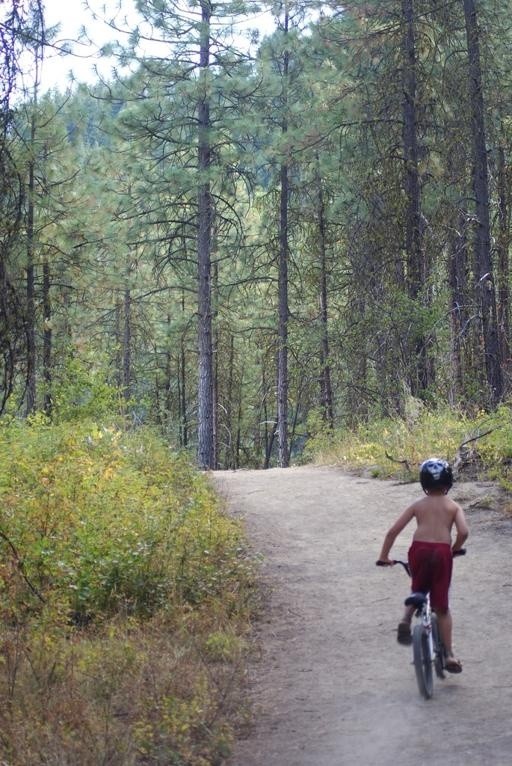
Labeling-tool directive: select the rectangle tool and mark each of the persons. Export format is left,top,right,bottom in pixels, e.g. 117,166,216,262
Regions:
379,457,469,674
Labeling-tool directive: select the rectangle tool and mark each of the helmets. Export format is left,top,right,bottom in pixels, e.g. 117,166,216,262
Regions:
419,458,453,491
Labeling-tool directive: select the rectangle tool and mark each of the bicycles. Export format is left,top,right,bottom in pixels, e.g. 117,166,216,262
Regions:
373,546,471,701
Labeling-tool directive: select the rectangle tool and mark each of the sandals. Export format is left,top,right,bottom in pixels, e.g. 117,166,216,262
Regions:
397,616,411,644
446,651,462,673
